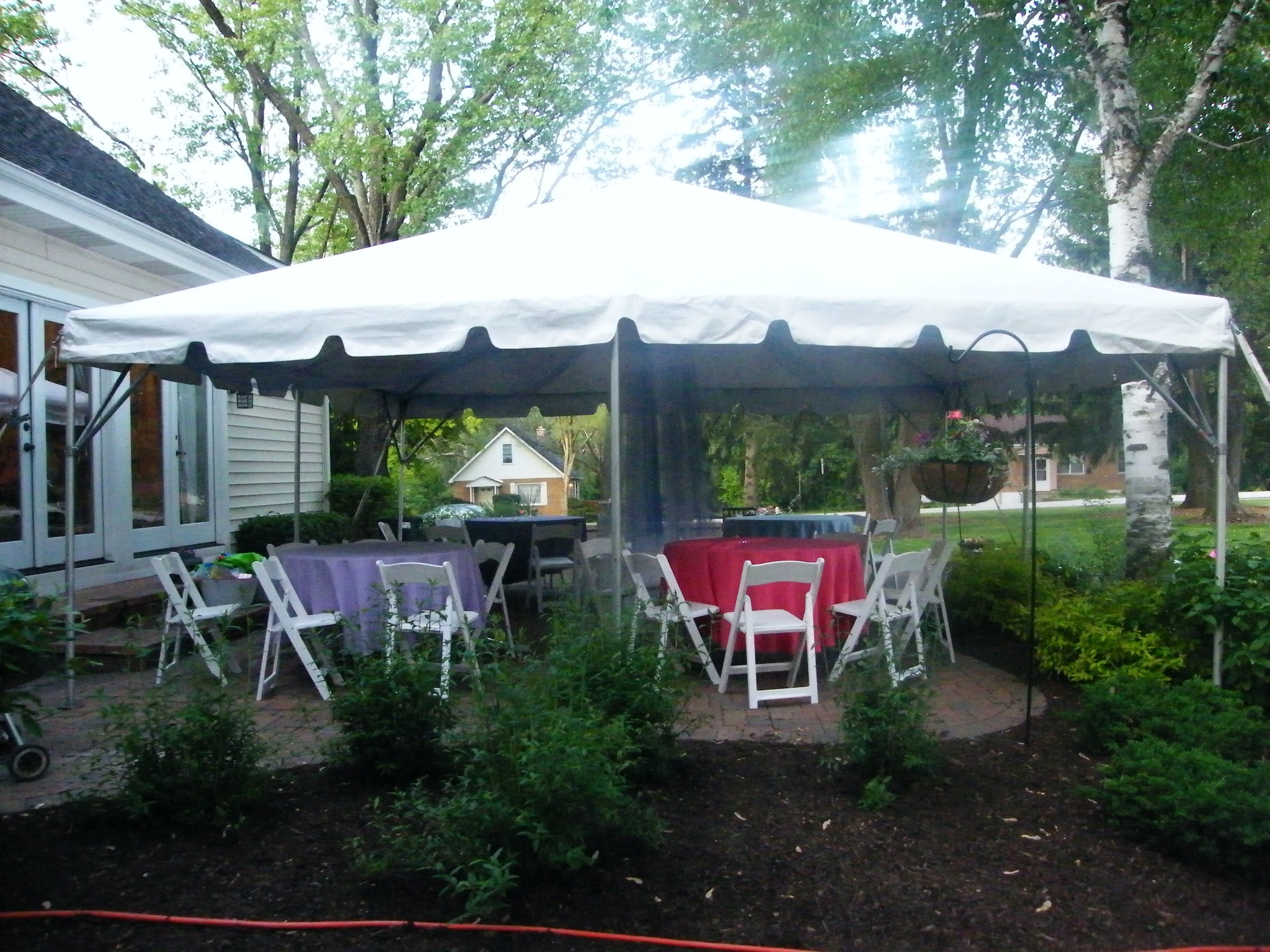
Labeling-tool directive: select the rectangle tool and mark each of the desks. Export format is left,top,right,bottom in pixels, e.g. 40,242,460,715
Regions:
272,542,488,656
464,515,588,587
660,537,871,657
721,514,855,539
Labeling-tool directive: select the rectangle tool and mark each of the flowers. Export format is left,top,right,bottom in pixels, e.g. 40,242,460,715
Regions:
874,409,1021,490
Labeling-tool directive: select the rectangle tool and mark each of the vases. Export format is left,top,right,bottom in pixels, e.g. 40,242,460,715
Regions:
909,459,1011,504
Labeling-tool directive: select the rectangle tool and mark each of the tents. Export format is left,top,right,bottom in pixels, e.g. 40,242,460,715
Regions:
52,168,1243,710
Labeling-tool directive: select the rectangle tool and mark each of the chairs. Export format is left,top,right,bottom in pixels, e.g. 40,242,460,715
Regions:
573,534,720,693
150,552,243,687
524,524,584,612
717,557,825,710
251,517,516,706
826,514,955,689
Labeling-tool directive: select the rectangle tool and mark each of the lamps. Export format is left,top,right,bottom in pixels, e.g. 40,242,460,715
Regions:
227,389,254,410
